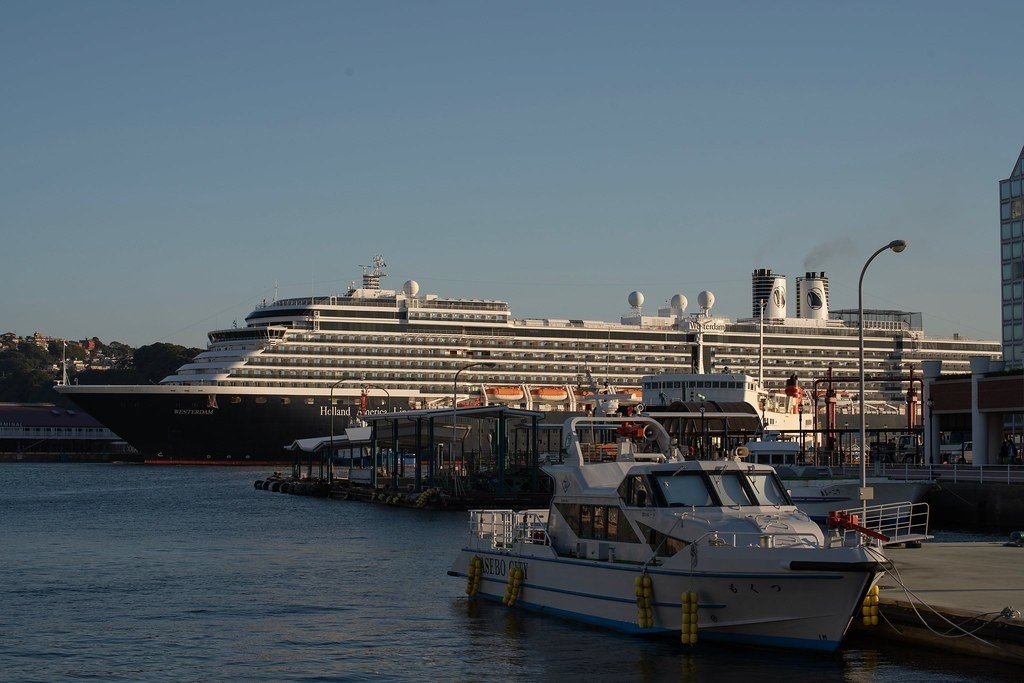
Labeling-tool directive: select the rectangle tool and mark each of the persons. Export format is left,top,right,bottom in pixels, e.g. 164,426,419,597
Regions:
659,390,668,404
798,437,1018,466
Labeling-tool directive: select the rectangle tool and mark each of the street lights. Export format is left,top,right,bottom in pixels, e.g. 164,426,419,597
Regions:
858,240,907,529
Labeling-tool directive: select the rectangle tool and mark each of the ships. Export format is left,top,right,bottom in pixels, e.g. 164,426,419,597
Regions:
50,250,1001,467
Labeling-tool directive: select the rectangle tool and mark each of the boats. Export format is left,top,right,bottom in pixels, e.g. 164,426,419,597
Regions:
446,457,895,655
282,360,941,545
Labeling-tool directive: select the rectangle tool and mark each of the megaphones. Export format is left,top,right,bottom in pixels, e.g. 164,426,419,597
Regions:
643,424,659,440
733,447,749,457
